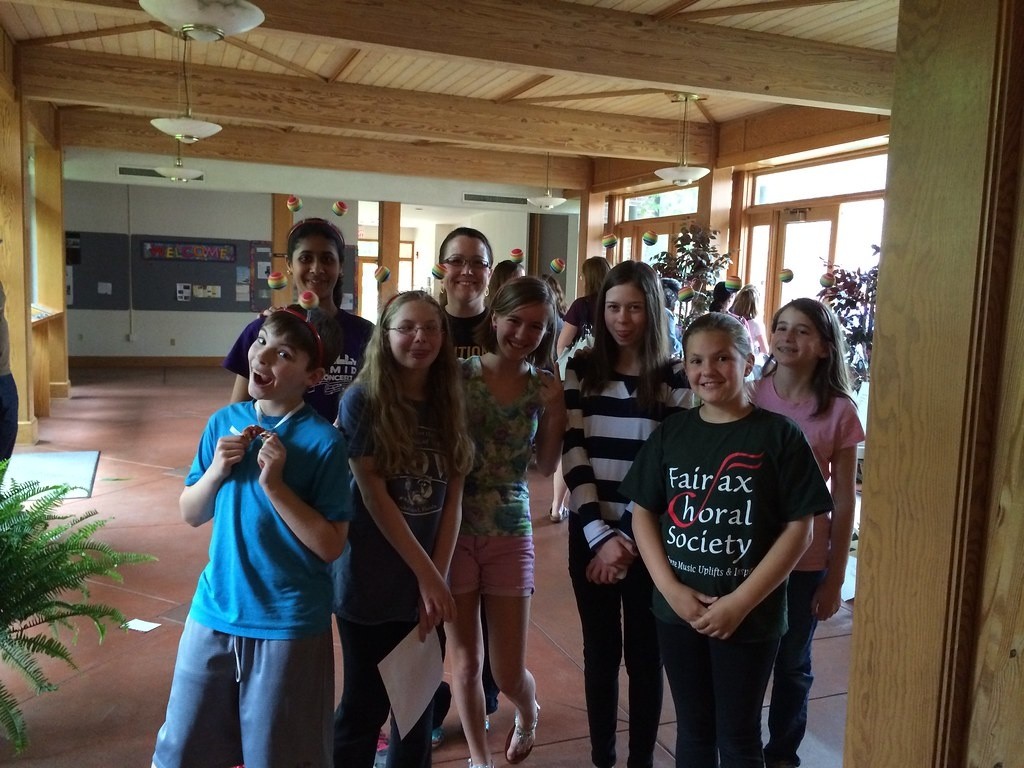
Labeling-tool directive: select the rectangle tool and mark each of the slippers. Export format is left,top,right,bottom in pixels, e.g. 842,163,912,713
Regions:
548,506,569,522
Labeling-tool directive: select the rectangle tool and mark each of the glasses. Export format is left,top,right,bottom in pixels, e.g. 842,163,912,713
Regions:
388,322,444,337
578,274,584,281
442,254,490,270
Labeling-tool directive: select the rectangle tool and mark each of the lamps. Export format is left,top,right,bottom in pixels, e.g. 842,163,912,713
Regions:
526,153,567,209
139,0,265,43
654,92,711,186
150,37,222,143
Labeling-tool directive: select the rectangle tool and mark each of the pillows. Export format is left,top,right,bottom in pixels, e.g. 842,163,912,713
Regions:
155,138,204,182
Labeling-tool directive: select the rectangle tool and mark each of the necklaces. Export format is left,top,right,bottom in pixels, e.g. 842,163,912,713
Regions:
241,399,306,447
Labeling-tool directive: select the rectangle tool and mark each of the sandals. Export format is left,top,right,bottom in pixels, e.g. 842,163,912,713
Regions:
504,699,540,765
467,758,495,768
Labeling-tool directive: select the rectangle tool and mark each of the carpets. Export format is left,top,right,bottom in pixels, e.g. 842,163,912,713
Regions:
0,451,100,502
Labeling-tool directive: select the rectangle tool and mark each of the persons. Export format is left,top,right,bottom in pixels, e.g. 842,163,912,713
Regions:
618,313,837,768
145,219,864,768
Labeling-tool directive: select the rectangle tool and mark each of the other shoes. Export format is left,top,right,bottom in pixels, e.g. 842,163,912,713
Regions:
432,723,446,750
461,714,490,741
373,728,390,768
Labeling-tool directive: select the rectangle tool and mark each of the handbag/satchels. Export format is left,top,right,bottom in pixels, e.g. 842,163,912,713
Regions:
555,296,596,382
